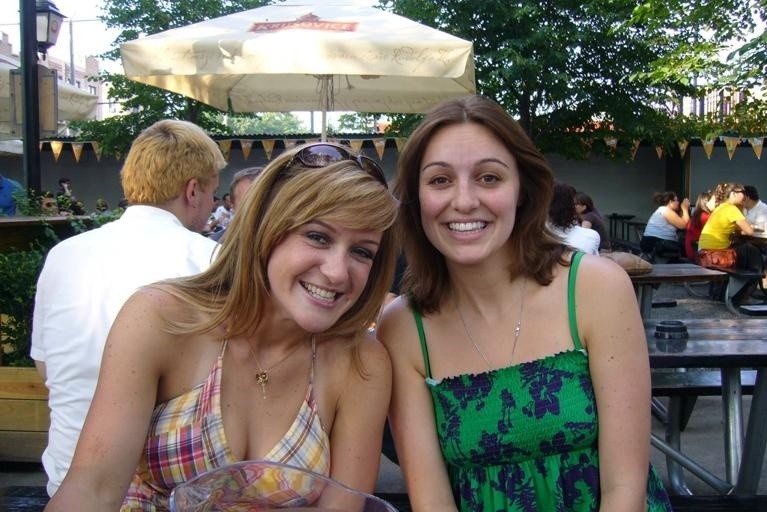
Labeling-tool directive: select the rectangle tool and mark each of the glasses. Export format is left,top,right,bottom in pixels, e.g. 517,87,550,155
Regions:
671,199,679,202
734,189,747,195
277,144,391,192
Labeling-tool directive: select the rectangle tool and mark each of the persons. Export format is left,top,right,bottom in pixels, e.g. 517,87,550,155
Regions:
213,196,232,229
31,120,227,511
39,190,58,218
374,96,672,512
90,197,109,219
643,184,763,285
58,176,81,216
544,178,613,255
230,169,265,209
44,144,393,511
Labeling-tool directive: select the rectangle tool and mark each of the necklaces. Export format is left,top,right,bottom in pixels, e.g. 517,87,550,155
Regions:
226,277,322,398
444,264,539,392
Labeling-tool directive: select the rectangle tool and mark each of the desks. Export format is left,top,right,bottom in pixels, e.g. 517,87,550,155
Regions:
642,319,766,511
612,262,727,319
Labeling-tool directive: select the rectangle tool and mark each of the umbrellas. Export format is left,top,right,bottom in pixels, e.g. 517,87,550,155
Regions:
116,2,478,142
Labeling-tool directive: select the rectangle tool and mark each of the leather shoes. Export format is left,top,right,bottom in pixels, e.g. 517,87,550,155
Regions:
742,297,762,305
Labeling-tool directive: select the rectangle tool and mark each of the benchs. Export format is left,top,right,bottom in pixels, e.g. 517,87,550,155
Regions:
613,238,765,302
649,367,760,496
0,367,54,512
